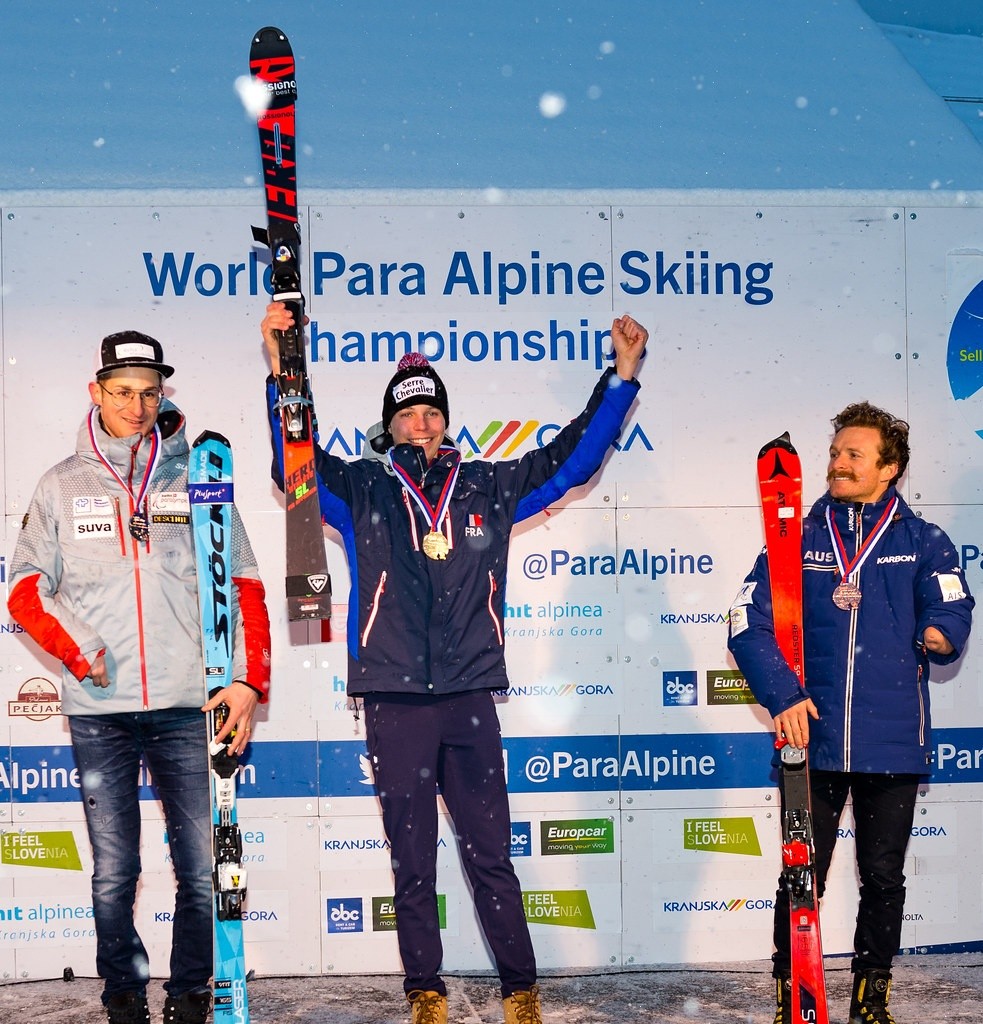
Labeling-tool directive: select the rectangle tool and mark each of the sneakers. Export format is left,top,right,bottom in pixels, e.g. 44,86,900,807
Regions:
406,989,447,1024
502,985,542,1024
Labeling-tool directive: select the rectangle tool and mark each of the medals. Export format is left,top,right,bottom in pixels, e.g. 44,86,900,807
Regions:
421,531,450,561
129,514,150,541
831,583,862,612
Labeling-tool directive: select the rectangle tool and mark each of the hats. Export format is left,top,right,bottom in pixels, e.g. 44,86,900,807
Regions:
380,352,449,434
94,330,174,383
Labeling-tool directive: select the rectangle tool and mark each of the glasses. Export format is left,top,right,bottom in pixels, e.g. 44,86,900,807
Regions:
96,381,162,408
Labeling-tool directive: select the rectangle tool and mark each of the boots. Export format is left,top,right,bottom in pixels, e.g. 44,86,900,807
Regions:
105,990,150,1024
847,968,895,1024
160,992,214,1024
772,976,790,1024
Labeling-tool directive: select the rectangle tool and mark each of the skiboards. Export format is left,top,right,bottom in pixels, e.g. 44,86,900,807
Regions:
248,24,333,650
755,438,832,1024
186,429,251,1024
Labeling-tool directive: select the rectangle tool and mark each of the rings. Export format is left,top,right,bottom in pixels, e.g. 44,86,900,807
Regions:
245,728,252,731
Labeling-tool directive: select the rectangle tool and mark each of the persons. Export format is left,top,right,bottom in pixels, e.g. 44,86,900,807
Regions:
726,401,974,1024
7,330,270,1024
260,302,650,1024
